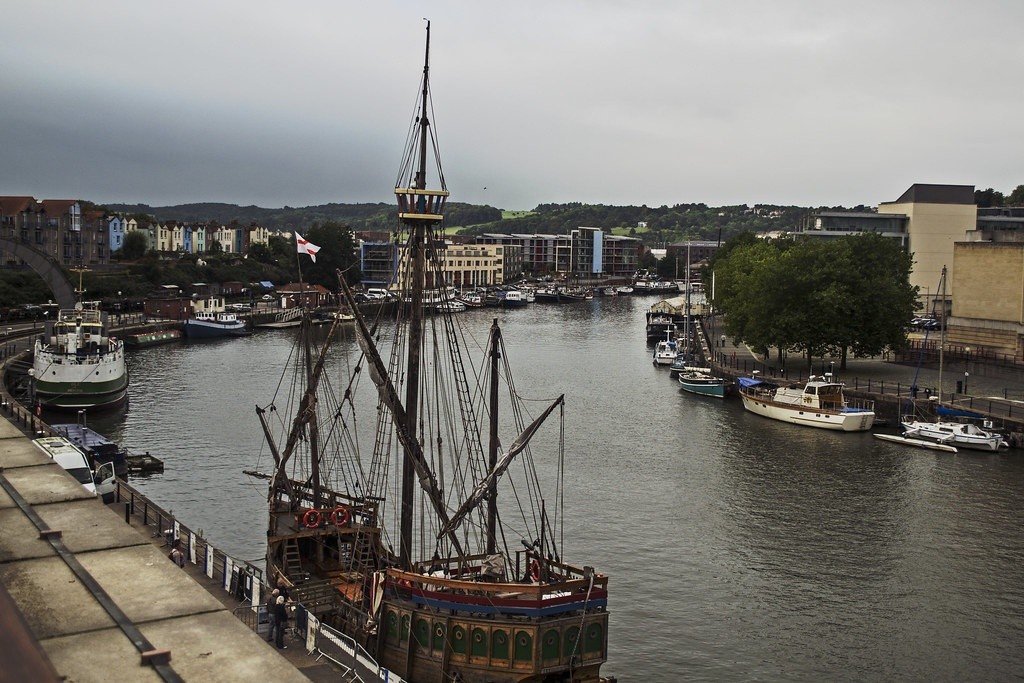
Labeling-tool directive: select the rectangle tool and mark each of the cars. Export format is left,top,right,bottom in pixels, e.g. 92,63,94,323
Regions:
353,293,376,301
910,316,946,330
226,303,251,312
470,285,521,293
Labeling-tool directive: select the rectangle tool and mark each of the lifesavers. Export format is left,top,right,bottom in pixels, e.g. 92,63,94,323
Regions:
530,559,541,581
331,508,349,526
303,509,321,528
37,407,41,416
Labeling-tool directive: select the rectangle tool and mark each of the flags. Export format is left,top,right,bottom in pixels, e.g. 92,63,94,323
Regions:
295,231,321,263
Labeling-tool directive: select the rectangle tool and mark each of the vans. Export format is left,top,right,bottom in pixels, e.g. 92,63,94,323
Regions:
262,295,272,300
35,436,117,500
368,288,392,300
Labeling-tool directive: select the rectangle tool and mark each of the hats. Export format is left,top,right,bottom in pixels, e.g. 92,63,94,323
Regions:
275,595,284,605
271,589,280,597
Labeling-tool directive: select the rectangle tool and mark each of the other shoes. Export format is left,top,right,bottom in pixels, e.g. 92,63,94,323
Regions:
266,639,274,642
276,645,288,649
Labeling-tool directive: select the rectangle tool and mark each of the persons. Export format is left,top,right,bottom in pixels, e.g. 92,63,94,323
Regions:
720,333,727,348
913,385,919,399
266,578,294,649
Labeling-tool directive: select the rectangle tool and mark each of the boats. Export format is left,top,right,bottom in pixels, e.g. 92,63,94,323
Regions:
51,409,129,478
646,296,680,364
534,276,684,300
737,372,876,431
403,285,466,313
455,291,536,308
677,371,734,397
29,263,129,410
320,311,356,321
182,296,248,341
124,328,184,348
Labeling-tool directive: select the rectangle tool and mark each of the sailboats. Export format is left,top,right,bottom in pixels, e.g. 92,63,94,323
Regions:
670,242,694,377
901,263,1005,452
240,19,612,683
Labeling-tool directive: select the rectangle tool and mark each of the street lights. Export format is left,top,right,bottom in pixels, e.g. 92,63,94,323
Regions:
965,347,970,394
922,287,928,318
48,300,52,319
117,291,122,325
27,369,36,430
